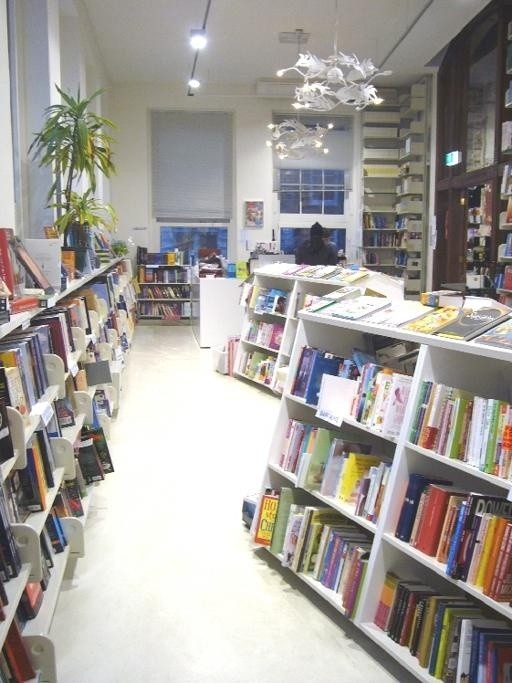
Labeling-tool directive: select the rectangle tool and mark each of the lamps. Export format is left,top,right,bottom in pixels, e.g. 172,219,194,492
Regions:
265,28,335,160
276,0,393,113
189,29,207,51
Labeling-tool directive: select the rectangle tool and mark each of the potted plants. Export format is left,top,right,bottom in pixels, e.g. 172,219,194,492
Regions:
27,81,119,279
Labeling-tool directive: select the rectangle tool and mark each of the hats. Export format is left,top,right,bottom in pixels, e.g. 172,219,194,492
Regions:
310,222,324,236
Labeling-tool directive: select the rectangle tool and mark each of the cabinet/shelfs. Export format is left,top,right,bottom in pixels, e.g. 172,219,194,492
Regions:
232,263,405,396
249,286,512,683
424,0,512,311
354,87,399,276
395,76,431,300
0,256,136,683
136,252,199,320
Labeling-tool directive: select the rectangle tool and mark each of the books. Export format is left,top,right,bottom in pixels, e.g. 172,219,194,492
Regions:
135,246,191,317
250,284,511,682
1,226,143,683
236,262,373,395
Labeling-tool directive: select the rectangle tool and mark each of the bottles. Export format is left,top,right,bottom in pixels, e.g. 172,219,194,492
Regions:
236,260,247,279
228,263,236,278
